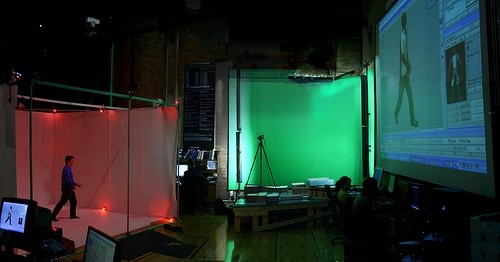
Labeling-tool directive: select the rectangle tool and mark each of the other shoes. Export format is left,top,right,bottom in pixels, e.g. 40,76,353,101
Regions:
70,215,80,218
50,215,59,221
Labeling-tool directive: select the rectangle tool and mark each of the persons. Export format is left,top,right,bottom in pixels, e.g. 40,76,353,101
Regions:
393,13,418,126
51,154,82,222
336,176,352,204
4,206,12,225
448,47,465,101
353,177,397,262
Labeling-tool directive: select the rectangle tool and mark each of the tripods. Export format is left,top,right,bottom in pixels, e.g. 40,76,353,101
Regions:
245,141,278,191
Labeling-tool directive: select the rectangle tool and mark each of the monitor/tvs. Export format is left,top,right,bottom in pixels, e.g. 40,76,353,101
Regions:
387,174,397,193
407,183,424,212
373,168,383,188
0,197,52,238
82,225,121,262
176,164,188,177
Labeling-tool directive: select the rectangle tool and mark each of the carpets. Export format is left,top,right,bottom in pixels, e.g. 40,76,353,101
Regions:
119,230,176,260
157,242,198,257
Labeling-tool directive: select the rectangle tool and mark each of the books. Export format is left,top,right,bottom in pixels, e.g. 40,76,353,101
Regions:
246,182,308,202
184,149,214,160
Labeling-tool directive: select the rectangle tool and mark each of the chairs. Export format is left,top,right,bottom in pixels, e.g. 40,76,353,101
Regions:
182,173,208,214
321,196,387,262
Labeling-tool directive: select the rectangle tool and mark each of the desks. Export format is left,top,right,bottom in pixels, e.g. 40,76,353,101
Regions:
233,198,329,232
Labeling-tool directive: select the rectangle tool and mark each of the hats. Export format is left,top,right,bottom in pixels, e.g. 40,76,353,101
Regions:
362,177,377,189
